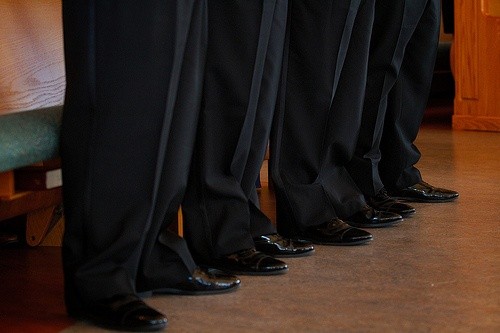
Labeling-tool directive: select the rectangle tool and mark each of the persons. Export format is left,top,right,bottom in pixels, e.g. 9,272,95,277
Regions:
345,0,461,218
61,0,241,332
269,0,404,246
181,0,315,275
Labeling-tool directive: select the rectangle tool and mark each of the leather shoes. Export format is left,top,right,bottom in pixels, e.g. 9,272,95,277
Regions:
338,205,404,227
254,233,316,257
388,180,460,202
369,189,416,216
149,264,241,294
69,299,167,331
288,215,375,246
198,247,290,275
